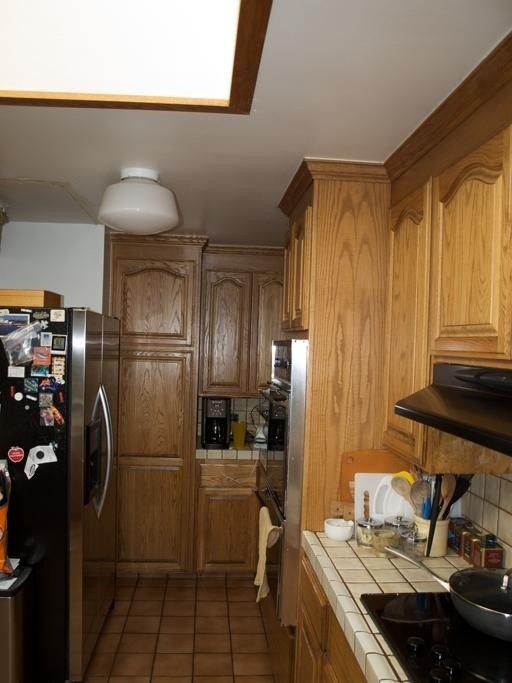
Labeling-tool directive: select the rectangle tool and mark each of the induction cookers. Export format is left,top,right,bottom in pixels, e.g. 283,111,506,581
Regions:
358,592,510,682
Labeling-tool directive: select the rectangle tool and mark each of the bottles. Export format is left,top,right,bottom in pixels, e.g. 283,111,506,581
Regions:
358,516,425,560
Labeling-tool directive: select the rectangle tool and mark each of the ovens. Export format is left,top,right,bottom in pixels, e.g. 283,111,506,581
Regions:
254,338,307,628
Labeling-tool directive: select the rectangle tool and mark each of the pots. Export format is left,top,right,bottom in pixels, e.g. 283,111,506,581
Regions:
382,542,511,641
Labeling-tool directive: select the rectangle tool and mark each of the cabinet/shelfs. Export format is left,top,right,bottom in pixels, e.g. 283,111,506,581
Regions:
382,101,512,478
196,460,257,577
95,226,214,577
420,29,512,370
277,153,390,331
284,546,367,681
198,242,285,396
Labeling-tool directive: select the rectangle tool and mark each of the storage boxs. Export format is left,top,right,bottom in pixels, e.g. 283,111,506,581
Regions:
1,287,62,310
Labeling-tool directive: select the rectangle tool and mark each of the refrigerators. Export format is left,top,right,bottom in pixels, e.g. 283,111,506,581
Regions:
0,306,119,676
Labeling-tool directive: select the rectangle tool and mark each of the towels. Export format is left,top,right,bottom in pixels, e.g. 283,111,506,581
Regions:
249,505,281,602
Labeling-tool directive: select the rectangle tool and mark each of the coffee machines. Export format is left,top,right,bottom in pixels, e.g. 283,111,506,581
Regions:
201,395,232,448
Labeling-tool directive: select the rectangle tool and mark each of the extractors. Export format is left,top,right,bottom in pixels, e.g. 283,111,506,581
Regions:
392,361,511,454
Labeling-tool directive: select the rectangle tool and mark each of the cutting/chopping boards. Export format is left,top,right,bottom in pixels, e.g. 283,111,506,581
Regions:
355,471,463,537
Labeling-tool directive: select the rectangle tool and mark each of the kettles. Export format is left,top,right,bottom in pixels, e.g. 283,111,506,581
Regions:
371,474,414,518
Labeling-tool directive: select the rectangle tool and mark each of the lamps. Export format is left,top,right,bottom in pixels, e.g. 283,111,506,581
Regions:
97,165,182,235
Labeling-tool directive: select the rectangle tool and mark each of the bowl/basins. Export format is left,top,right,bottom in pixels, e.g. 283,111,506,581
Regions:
322,517,355,540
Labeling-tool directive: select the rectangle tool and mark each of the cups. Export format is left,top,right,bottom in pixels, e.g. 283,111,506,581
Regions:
231,420,246,448
413,514,450,556
